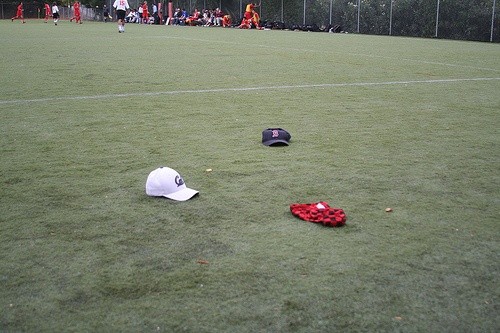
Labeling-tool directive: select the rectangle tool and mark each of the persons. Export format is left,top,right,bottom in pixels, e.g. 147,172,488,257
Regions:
70,1,83,24
165,7,233,28
44,2,61,26
104,0,163,33
11,2,25,24
235,3,266,30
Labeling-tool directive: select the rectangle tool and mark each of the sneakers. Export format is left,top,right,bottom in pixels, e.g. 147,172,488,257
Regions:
118,27,125,33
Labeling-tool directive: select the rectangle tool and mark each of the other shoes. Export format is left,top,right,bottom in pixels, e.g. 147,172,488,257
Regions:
11,18,14,22
55,24,57,25
43,21,48,23
80,22,83,24
22,22,26,23
70,18,72,22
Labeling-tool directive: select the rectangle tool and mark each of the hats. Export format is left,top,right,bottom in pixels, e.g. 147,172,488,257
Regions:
146,167,200,201
262,127,291,147
